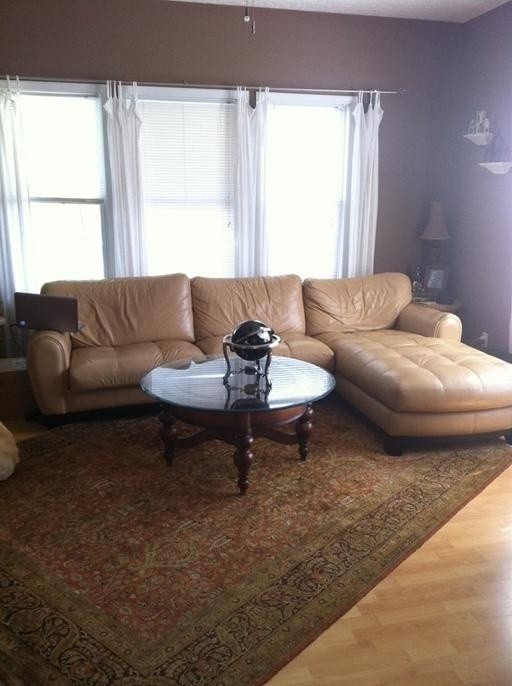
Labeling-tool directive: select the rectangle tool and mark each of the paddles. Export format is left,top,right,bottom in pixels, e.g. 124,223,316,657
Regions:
0,406,512,685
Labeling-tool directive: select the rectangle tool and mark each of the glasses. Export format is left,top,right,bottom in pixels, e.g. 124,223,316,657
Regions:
415,202,451,262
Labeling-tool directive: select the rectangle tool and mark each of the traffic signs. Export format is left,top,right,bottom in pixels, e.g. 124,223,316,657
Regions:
480,330,488,349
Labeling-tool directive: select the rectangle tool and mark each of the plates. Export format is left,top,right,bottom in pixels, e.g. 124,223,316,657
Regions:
461,131,511,177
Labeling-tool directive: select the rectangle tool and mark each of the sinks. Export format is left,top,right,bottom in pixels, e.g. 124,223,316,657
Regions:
188,275,335,384
303,268,512,455
25,270,208,432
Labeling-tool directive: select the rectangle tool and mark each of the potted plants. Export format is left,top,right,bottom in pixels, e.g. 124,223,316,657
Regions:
419,263,449,293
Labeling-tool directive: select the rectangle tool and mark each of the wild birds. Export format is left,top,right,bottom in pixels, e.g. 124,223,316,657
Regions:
14,292,85,332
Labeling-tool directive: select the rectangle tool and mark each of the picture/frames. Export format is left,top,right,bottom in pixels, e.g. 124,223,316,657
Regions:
407,293,465,321
